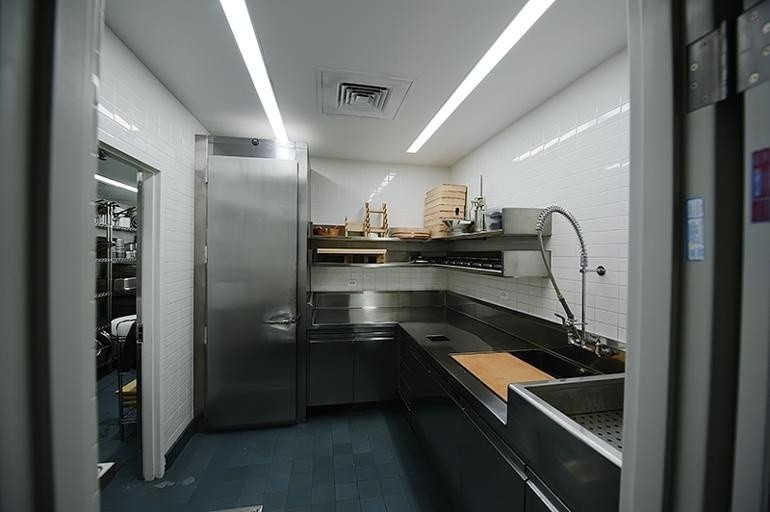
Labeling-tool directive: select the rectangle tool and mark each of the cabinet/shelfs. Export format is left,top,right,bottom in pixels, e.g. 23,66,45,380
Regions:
308,337,397,407
111,314,138,442
94,205,137,381
396,334,428,424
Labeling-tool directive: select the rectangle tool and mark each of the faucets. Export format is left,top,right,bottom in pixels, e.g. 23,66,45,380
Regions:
536,204,620,358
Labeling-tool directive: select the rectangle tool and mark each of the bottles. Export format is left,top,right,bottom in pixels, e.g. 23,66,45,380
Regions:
112,237,137,258
113,213,119,226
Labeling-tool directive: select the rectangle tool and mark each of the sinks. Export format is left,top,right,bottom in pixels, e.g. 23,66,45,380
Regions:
508,346,606,380
